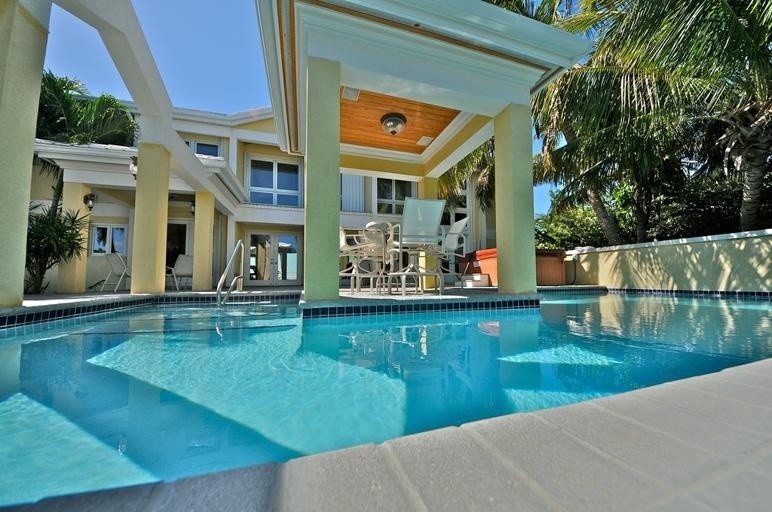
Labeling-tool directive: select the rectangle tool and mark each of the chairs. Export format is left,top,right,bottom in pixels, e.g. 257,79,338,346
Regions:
100,252,193,293
340,197,471,297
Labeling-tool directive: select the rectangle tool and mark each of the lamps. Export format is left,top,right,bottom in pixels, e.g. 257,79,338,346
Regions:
128,155,138,180
83,193,98,212
380,112,406,137
